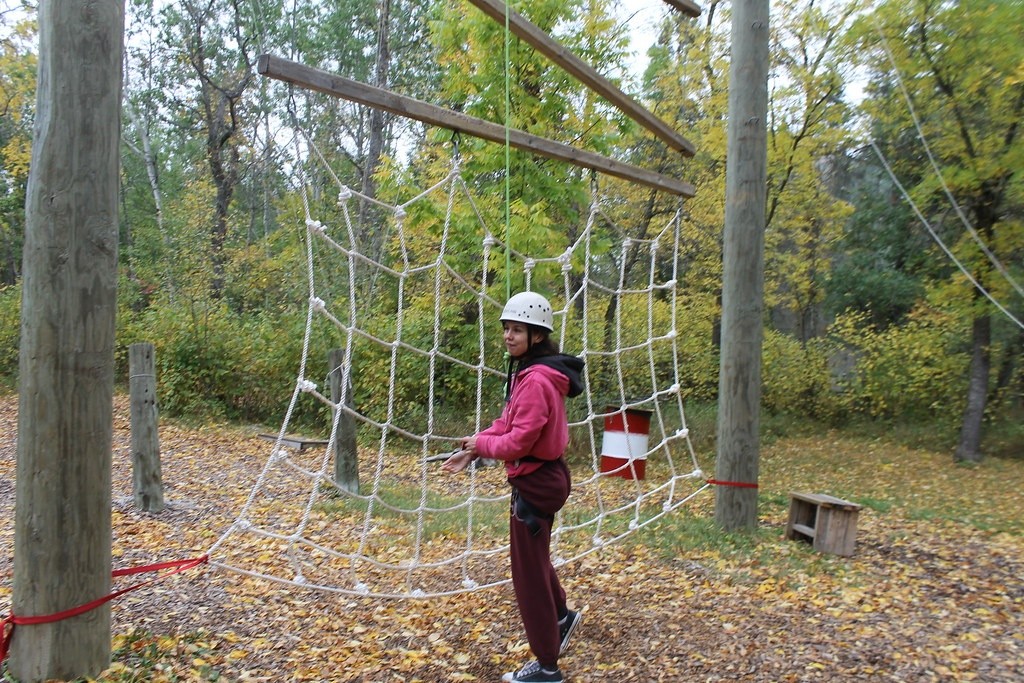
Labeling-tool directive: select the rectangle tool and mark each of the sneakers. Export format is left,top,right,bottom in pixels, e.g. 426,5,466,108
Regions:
502,661,564,683
557,610,581,657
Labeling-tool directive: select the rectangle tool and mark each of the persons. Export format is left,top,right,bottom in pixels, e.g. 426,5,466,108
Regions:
439,292,585,683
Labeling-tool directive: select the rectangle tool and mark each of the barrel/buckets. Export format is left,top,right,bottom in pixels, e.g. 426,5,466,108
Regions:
600,406,651,479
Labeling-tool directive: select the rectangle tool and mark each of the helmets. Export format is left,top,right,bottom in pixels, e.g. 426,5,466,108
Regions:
499,291,554,332
